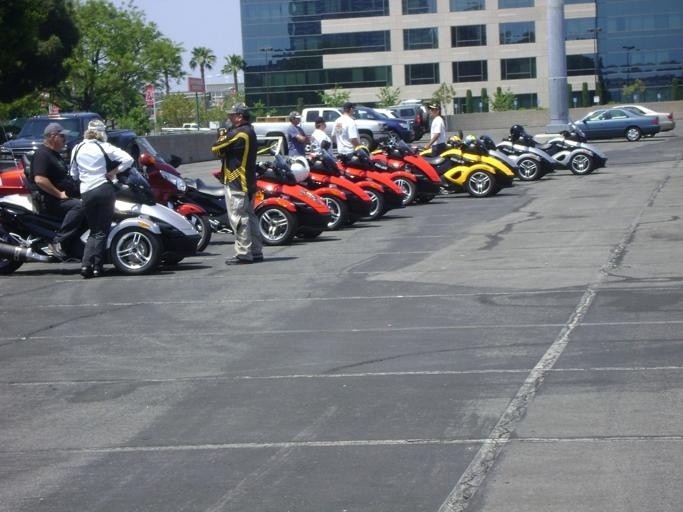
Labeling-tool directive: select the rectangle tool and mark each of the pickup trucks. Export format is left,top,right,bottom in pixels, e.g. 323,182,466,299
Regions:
250,108,388,156
1,112,139,170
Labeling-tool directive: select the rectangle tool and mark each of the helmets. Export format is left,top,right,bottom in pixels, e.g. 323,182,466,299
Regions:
510,125,525,137
288,155,312,184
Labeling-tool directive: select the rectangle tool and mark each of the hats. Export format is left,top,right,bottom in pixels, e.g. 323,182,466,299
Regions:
427,103,441,111
315,117,327,124
224,102,250,117
343,102,354,109
43,122,72,137
288,111,303,120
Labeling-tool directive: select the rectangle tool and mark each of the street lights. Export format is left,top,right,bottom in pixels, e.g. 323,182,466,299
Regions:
259,47,273,111
622,46,635,86
588,27,601,91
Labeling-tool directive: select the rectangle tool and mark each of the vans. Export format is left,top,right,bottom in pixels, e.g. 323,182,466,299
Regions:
183,123,199,128
332,99,429,143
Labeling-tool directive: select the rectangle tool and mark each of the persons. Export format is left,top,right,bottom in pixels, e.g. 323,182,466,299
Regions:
312,117,335,154
422,101,446,153
68,118,134,276
288,110,313,156
332,101,362,154
212,103,265,265
29,121,84,261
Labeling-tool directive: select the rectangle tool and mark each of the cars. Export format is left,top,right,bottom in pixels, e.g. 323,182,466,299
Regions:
568,104,675,142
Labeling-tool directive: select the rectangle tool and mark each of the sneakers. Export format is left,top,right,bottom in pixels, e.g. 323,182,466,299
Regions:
48,242,69,258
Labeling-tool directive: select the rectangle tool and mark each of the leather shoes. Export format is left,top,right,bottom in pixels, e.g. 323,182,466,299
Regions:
254,253,263,262
93,265,105,277
80,266,94,278
225,256,253,265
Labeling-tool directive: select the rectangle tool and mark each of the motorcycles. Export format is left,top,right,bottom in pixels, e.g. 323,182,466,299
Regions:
411,125,607,197
183,134,441,246
0,153,212,275
